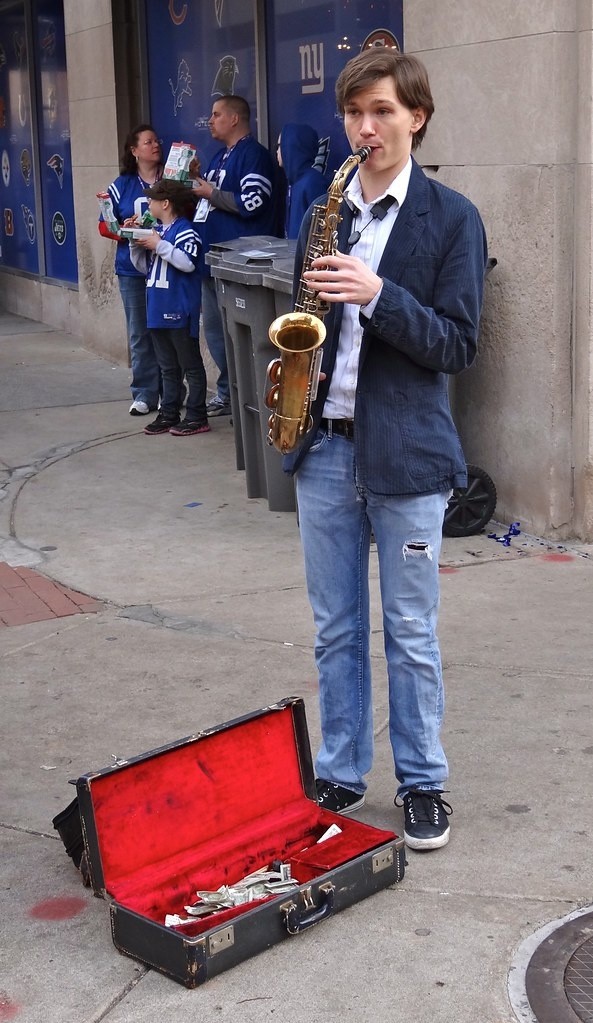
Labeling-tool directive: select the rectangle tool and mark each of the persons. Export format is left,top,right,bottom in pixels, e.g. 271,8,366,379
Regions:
276,124,329,239
98,125,186,414
283,46,488,850
123,179,210,435
188,95,283,427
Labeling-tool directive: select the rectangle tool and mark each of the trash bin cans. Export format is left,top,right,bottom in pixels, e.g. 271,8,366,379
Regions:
205,235,298,513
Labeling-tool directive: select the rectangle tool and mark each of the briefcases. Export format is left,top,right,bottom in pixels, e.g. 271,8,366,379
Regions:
68,695,407,989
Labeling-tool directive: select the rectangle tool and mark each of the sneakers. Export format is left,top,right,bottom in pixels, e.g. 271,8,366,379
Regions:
130,400,162,416
315,777,366,816
393,787,455,850
143,412,183,435
169,415,210,437
206,395,231,418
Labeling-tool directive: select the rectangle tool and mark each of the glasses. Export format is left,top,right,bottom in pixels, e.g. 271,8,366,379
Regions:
135,139,163,148
276,143,281,150
146,196,152,202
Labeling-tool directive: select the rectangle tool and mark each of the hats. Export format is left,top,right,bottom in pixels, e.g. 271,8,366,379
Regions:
143,179,183,200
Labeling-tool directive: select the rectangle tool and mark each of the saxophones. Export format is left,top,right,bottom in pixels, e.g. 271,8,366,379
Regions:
264,145,371,455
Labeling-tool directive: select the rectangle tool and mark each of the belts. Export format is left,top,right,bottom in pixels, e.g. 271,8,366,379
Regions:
319,418,356,441
